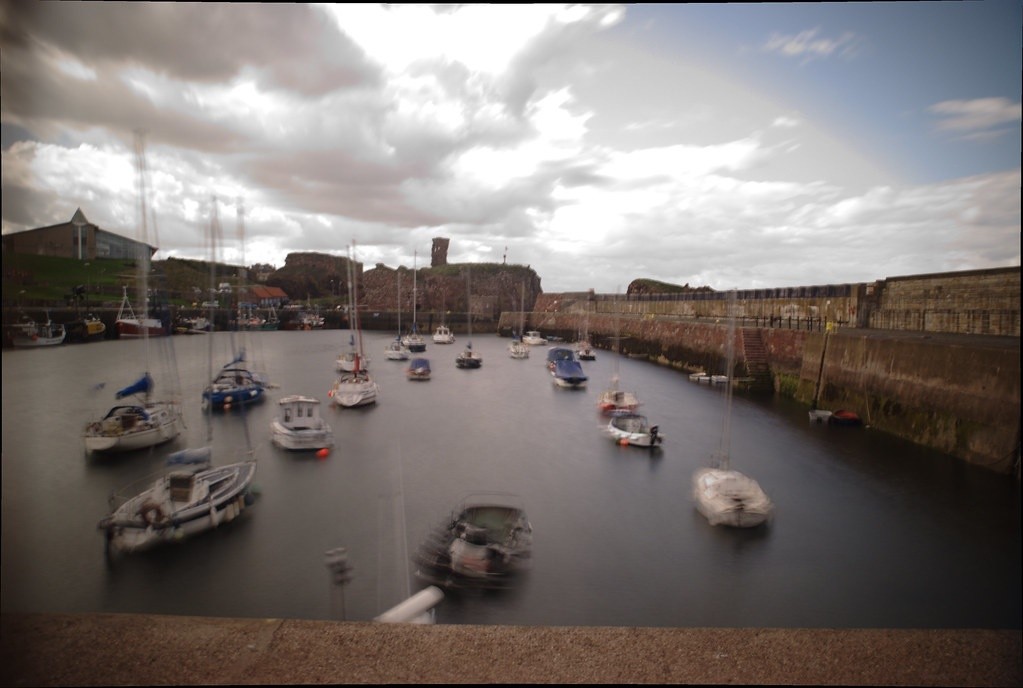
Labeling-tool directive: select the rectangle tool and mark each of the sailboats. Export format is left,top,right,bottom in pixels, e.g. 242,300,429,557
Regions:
430,288,455,345
331,236,378,408
335,243,371,373
402,249,426,352
201,190,271,412
99,190,258,573
596,283,638,417
578,284,596,360
454,262,483,369
62,261,119,343
691,286,773,528
386,271,411,360
509,278,530,360
80,129,187,464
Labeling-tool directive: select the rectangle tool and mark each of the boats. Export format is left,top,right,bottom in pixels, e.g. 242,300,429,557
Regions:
270,395,335,455
521,330,548,345
169,299,368,335
115,272,166,337
411,491,534,597
7,320,68,347
809,409,861,425
546,347,588,389
406,359,431,380
608,410,662,447
690,372,728,382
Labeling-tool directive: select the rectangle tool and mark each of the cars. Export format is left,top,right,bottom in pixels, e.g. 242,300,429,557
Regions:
238,302,257,309
202,301,221,309
284,302,305,310
